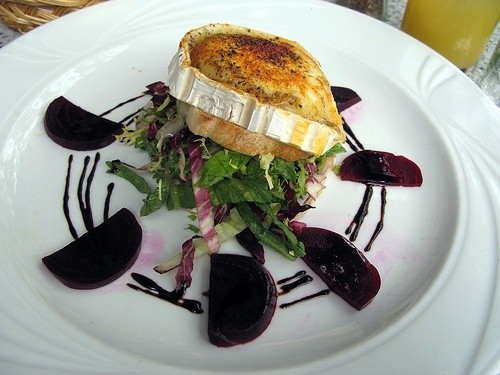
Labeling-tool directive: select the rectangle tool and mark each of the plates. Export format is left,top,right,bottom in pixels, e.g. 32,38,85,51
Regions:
0,0,500,374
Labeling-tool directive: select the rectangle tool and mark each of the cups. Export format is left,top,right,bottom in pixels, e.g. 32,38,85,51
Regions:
402,0,499,70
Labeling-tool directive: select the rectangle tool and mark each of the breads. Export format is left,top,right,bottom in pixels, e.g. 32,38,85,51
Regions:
169,27,347,162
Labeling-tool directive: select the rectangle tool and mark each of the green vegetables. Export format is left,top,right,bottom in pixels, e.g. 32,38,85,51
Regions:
105,89,348,262
